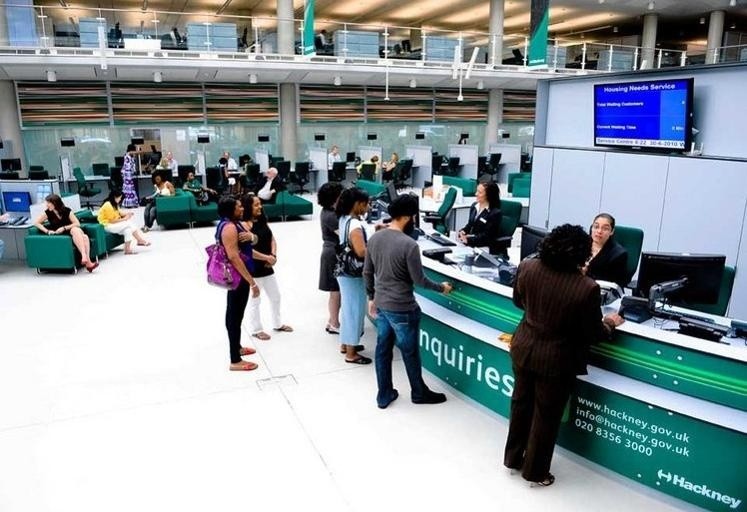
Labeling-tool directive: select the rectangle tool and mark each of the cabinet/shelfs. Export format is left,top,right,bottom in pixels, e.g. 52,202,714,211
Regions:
0,180,80,264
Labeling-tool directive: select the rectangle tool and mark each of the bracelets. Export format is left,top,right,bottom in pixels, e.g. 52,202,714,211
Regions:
250,232,254,242
271,252,277,258
46,229,49,235
250,282,257,289
62,224,67,232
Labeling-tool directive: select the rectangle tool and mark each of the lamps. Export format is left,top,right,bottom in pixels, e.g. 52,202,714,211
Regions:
152,71,162,82
249,73,258,83
476,80,484,89
333,76,342,85
47,70,56,81
408,78,416,87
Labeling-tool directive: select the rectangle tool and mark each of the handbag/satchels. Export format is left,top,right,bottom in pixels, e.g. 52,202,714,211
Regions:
205,221,248,290
333,218,368,278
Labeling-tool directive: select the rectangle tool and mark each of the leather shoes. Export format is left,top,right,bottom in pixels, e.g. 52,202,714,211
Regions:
87,262,100,272
378,389,398,409
413,391,446,404
80,260,88,265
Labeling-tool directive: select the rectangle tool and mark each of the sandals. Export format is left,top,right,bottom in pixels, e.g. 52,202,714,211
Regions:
329,326,340,334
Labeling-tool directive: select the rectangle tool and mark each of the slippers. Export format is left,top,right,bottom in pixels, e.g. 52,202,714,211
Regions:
125,250,137,254
252,333,271,340
274,325,293,332
341,344,365,354
137,241,151,245
345,356,372,364
239,347,256,356
230,361,258,371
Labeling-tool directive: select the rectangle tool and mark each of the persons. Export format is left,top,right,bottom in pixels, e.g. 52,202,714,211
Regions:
361,193,454,410
97,189,153,256
355,189,391,337
120,144,140,209
139,145,399,233
316,29,331,55
502,221,626,488
32,194,99,274
240,193,294,341
334,186,372,365
213,197,261,371
584,211,629,287
457,179,503,255
316,182,344,336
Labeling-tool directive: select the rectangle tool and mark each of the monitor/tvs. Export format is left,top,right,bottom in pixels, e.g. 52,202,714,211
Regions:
636,252,726,304
594,77,694,154
385,180,398,204
520,225,552,261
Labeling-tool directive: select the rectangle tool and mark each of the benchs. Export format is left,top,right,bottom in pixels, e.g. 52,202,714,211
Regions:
155,189,314,229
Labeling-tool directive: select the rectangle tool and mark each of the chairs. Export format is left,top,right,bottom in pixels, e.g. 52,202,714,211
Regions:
589,224,736,317
513,49,524,65
495,201,521,260
110,29,121,48
379,40,421,59
315,39,332,55
25,208,123,275
422,187,456,236
237,28,248,51
171,27,186,48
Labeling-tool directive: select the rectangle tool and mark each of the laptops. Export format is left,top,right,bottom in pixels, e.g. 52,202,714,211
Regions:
3,191,31,212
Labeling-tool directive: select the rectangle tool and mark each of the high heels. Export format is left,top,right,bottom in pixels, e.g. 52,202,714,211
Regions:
528,473,555,488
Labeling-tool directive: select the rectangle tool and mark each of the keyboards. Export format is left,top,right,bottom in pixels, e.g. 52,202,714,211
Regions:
426,234,457,246
654,307,714,324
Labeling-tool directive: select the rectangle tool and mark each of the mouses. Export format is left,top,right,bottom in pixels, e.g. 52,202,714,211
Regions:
431,233,440,236
